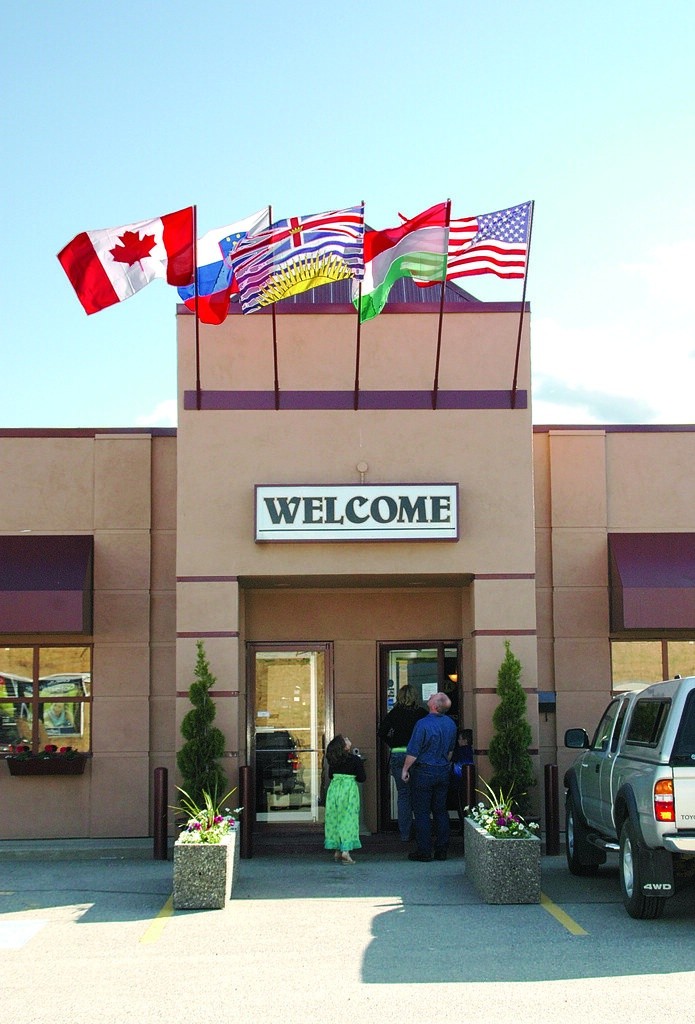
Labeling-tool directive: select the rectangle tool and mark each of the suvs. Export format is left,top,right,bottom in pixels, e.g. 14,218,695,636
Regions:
255,727,305,797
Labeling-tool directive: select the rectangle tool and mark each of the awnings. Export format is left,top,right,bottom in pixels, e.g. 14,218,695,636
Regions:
609,533,695,632
0,536,94,635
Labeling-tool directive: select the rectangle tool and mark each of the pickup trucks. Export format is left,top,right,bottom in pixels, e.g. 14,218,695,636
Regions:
561,675,695,918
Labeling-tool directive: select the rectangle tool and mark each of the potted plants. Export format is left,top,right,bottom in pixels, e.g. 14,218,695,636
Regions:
489,638,541,824
175,641,229,838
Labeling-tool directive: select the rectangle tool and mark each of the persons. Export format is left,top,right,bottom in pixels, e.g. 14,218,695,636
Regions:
43,703,77,729
454,729,473,817
402,692,456,862
380,685,429,842
324,735,366,864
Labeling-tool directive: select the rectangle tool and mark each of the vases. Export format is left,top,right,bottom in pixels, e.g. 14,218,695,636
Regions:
464,816,540,904
173,820,241,912
8,755,87,775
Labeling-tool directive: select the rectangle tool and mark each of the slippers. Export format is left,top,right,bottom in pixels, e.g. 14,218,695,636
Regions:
335,856,342,863
342,856,356,865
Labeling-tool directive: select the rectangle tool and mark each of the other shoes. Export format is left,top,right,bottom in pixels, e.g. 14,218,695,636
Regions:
433,850,447,861
408,850,432,861
401,832,414,842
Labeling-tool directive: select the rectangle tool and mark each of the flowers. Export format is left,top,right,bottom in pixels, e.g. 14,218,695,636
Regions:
166,776,245,845
10,745,79,760
464,774,541,838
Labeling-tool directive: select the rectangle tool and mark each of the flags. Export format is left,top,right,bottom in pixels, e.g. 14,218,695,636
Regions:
177,209,269,324
231,205,363,315
352,201,451,324
57,206,193,315
398,201,531,288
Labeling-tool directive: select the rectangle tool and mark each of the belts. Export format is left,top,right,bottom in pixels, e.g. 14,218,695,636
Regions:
391,747,407,753
